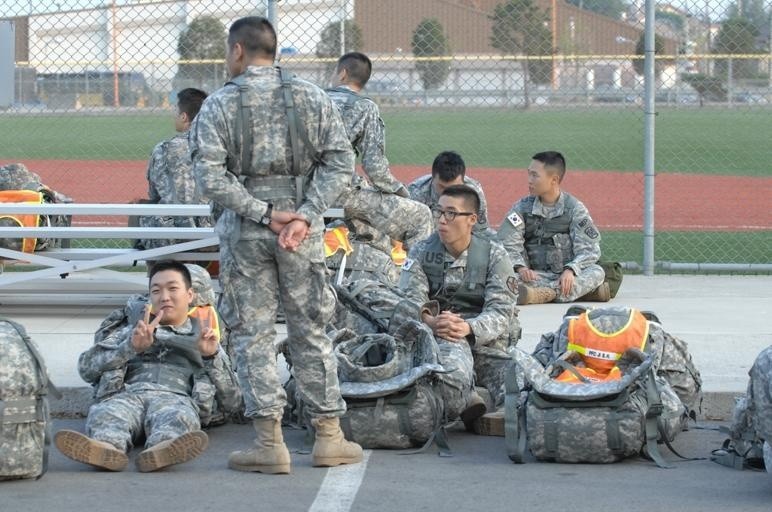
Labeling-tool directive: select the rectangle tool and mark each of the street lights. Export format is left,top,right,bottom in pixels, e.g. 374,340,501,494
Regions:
597,260,624,298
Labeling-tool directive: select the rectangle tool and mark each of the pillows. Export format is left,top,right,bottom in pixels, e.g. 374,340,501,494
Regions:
333,333,399,382
183,263,215,307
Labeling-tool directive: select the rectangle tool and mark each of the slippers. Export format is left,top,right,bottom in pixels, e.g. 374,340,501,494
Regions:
303,326,464,457
531,306,705,434
0,318,64,481
711,344,772,475
89,291,246,428
506,348,708,468
0,162,74,280
322,225,413,324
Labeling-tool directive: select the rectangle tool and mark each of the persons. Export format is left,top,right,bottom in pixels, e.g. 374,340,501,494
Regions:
318,51,433,264
404,149,486,230
497,150,612,306
51,263,221,471
185,16,364,472
400,185,517,416
136,87,223,265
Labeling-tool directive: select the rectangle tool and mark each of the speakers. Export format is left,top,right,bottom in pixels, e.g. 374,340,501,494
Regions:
228,419,290,474
582,282,610,302
310,416,363,467
134,430,208,473
53,429,128,472
517,285,557,305
465,407,505,436
460,393,487,421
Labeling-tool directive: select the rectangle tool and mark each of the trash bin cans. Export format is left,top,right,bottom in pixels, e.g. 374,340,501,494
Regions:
261,204,272,227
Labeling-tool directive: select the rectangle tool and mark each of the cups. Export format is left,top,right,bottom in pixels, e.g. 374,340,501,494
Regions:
432,208,471,222
